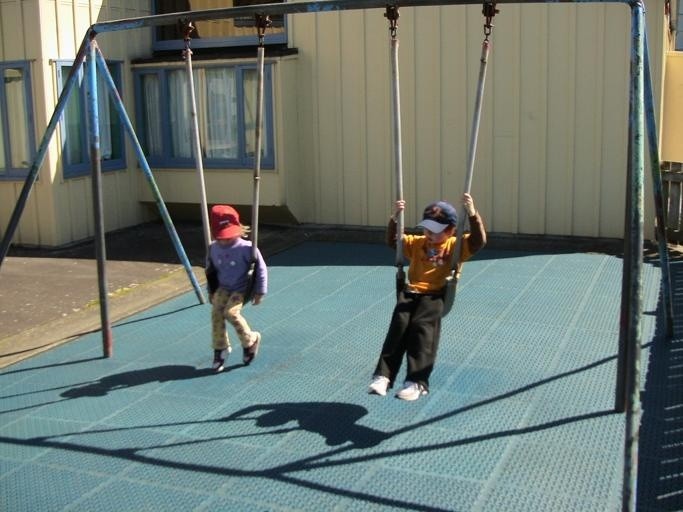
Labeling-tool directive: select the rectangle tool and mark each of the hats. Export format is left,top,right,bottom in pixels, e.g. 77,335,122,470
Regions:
416,200,458,234
209,204,243,240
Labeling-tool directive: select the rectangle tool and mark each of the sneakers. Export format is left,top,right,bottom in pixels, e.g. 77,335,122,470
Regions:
393,380,429,402
211,346,232,374
365,375,395,396
242,331,262,366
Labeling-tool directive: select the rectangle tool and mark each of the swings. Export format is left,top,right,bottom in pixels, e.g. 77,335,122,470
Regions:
384,3,499,316
178,16,272,305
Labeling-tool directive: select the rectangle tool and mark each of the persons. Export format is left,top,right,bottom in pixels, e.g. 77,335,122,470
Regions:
204,204,268,374
368,192,487,401
